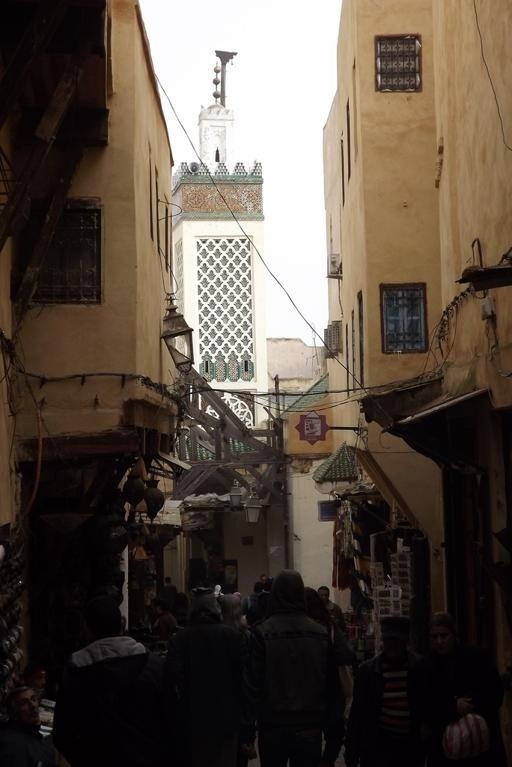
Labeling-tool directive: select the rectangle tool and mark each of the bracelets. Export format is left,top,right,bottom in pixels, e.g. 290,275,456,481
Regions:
0,557,25,679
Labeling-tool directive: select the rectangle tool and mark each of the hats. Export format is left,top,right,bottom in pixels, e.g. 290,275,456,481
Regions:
380,616,411,641
83,596,122,638
185,586,224,623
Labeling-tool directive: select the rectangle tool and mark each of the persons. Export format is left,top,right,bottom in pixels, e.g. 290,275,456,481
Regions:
0,685,56,767
317,588,345,632
406,612,507,762
343,617,426,762
55,567,274,765
303,585,345,763
238,569,332,762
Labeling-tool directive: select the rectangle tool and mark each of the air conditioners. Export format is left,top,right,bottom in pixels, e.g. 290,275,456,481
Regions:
321,321,344,357
327,253,342,278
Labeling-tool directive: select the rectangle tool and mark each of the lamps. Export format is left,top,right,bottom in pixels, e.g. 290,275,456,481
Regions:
122,455,145,509
143,465,164,527
229,478,248,511
240,486,264,527
154,241,195,377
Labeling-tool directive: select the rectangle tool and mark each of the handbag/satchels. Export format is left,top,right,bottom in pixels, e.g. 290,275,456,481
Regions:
441,712,491,760
229,623,268,702
